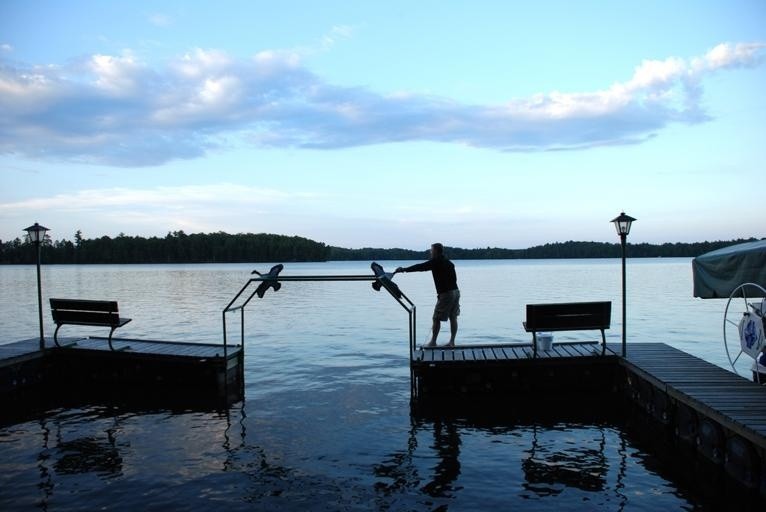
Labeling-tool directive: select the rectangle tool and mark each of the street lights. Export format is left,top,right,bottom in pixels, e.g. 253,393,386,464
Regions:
24,223,50,338
610,212,637,356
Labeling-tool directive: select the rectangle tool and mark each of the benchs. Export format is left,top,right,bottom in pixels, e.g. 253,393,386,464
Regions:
48,298,132,353
522,301,611,357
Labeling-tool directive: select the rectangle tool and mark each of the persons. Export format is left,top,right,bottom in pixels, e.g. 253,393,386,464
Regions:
396,244,462,346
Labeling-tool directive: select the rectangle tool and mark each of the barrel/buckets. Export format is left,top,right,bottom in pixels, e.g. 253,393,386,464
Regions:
536,333,554,350
